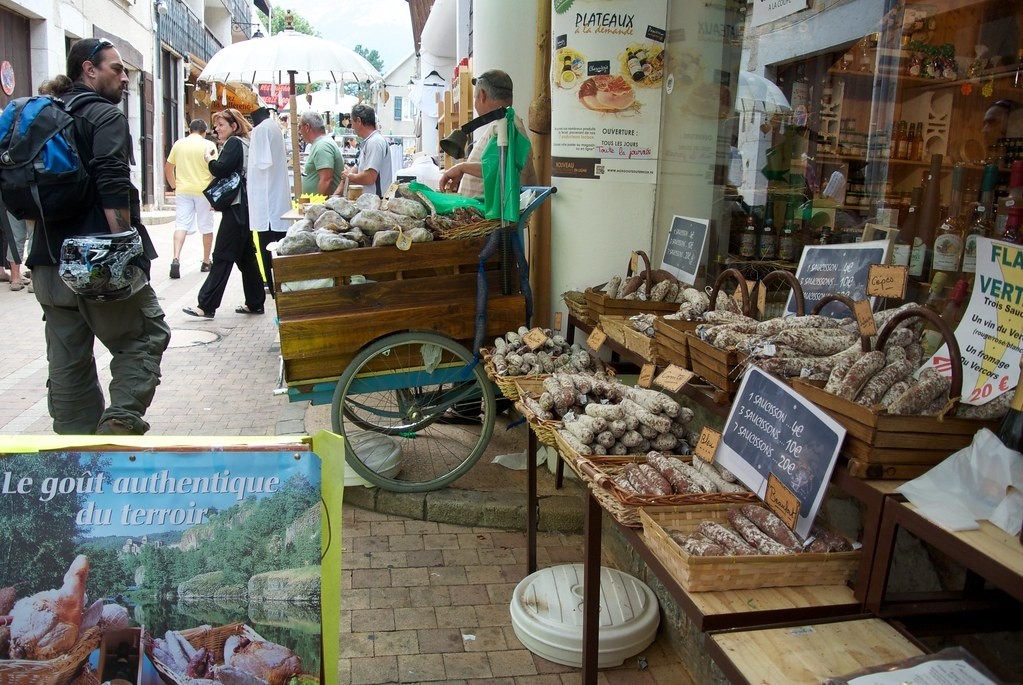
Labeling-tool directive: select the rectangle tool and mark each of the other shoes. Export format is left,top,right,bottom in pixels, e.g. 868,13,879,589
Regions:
235,305,264,313
0,260,33,293
170,258,180,278
96,419,137,435
182,307,216,318
496,400,514,413
202,261,212,271
437,415,482,425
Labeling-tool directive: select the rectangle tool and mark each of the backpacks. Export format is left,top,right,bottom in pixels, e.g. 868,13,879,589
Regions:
0,92,117,222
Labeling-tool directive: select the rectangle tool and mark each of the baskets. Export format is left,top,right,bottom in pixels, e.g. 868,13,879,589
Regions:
563,295,595,324
633,502,861,592
513,392,756,527
145,621,266,685
0,625,101,685
479,346,615,401
425,206,534,239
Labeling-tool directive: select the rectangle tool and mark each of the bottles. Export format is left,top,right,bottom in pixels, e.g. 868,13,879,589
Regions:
757,199,777,260
845,178,912,205
908,153,943,277
890,118,924,161
626,48,646,80
919,279,969,366
736,216,759,258
914,271,950,341
929,165,969,286
892,186,922,265
562,56,572,73
819,226,832,245
986,137,1023,245
777,201,796,260
960,162,998,293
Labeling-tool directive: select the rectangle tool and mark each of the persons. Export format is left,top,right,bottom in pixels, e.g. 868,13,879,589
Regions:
968,98,1023,232
25,37,170,463
0,68,536,318
726,147,743,195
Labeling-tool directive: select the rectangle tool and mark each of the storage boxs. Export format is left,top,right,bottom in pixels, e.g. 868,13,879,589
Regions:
272,224,529,383
687,326,737,403
651,312,707,370
736,344,786,389
786,368,1007,482
582,281,682,327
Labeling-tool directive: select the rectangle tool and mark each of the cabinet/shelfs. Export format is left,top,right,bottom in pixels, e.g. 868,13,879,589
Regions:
725,66,1023,303
437,67,473,171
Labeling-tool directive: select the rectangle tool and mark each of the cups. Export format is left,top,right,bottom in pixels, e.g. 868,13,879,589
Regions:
561,70,577,88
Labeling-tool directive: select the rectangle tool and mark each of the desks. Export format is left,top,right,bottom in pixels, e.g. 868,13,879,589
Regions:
511,308,1023,685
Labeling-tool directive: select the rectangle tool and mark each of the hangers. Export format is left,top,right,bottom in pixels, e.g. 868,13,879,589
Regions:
423,61,447,89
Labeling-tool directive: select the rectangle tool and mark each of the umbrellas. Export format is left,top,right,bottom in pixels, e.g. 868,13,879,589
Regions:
194,9,385,206
734,67,795,132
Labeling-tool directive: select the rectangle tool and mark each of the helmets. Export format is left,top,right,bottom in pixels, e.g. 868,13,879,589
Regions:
59,226,149,301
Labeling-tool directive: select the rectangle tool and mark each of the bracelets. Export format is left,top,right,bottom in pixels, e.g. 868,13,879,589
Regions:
173,188,176,191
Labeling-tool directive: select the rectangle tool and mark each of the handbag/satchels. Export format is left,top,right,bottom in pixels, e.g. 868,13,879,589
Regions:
202,171,242,211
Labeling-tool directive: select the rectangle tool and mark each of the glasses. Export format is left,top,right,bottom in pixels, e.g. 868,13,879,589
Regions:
88,38,113,60
471,76,490,84
225,109,236,121
298,125,305,130
